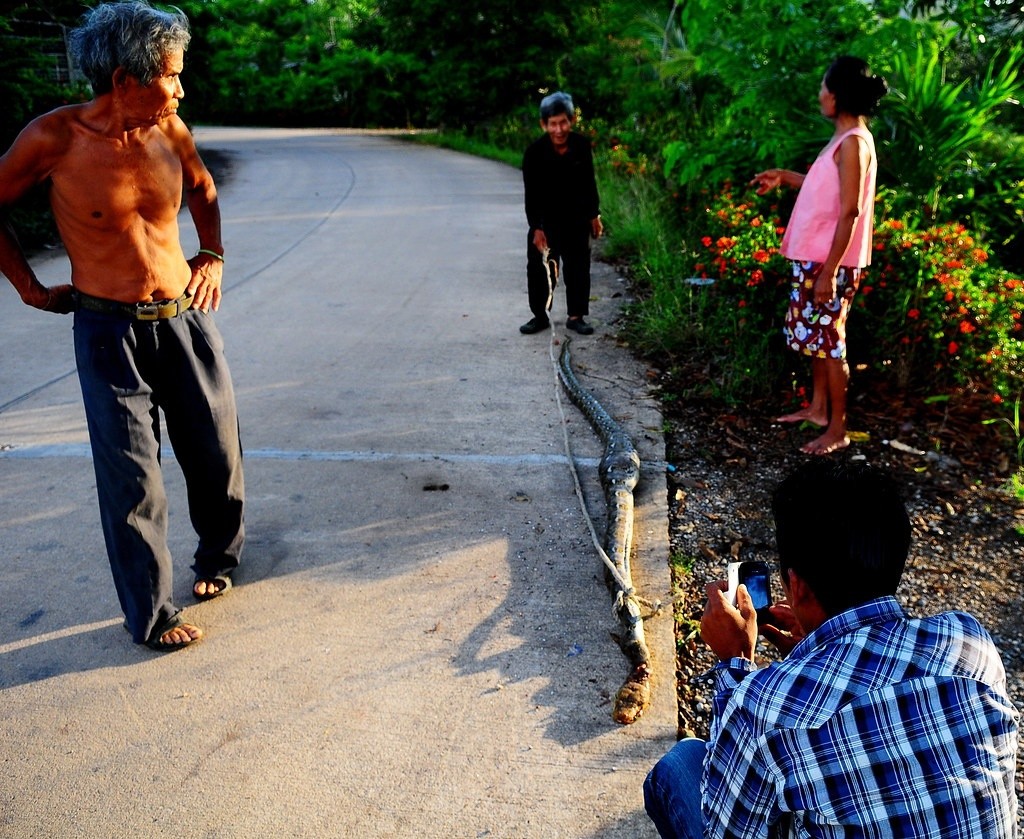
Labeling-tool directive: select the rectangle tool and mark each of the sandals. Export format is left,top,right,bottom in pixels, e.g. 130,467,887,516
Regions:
123,623,197,651
192,574,232,597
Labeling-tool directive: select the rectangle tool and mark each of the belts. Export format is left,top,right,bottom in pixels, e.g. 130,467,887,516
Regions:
70,291,194,320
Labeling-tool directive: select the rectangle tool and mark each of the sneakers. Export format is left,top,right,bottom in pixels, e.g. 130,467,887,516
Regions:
520,318,550,334
566,317,594,335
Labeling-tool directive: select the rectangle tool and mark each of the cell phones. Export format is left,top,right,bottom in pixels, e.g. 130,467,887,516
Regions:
738,561,775,625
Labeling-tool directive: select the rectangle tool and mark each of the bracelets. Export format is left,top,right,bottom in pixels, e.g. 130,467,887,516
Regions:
597,215,601,218
195,249,224,263
40,288,52,310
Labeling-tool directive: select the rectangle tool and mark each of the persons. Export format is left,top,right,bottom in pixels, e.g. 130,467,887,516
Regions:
520,94,603,337
751,56,889,453
0,0,246,650
643,451,1018,839
